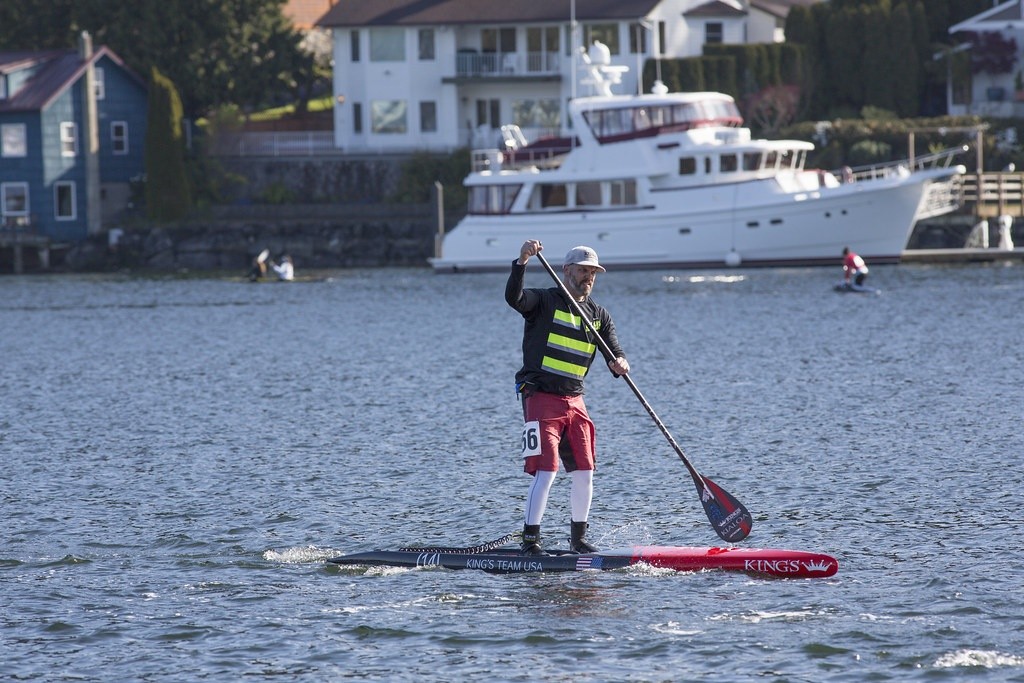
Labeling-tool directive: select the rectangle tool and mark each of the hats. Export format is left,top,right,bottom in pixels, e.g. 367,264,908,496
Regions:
562,245,606,273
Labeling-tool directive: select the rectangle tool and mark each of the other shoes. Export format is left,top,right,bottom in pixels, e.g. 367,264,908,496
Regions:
520,541,550,556
568,536,600,553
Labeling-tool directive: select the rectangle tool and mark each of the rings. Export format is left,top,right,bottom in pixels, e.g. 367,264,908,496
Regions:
528,246,531,249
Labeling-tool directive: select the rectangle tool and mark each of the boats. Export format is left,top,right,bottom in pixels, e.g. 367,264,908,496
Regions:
223,269,335,284
426,11,970,270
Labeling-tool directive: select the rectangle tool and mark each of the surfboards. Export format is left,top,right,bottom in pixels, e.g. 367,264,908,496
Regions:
323,540,841,582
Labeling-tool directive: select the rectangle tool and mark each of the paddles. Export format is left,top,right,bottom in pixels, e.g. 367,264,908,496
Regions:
245,248,271,277
534,239,756,543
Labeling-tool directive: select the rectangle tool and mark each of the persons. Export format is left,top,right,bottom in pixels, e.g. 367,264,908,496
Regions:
243,250,295,282
504,239,631,557
842,248,872,285
